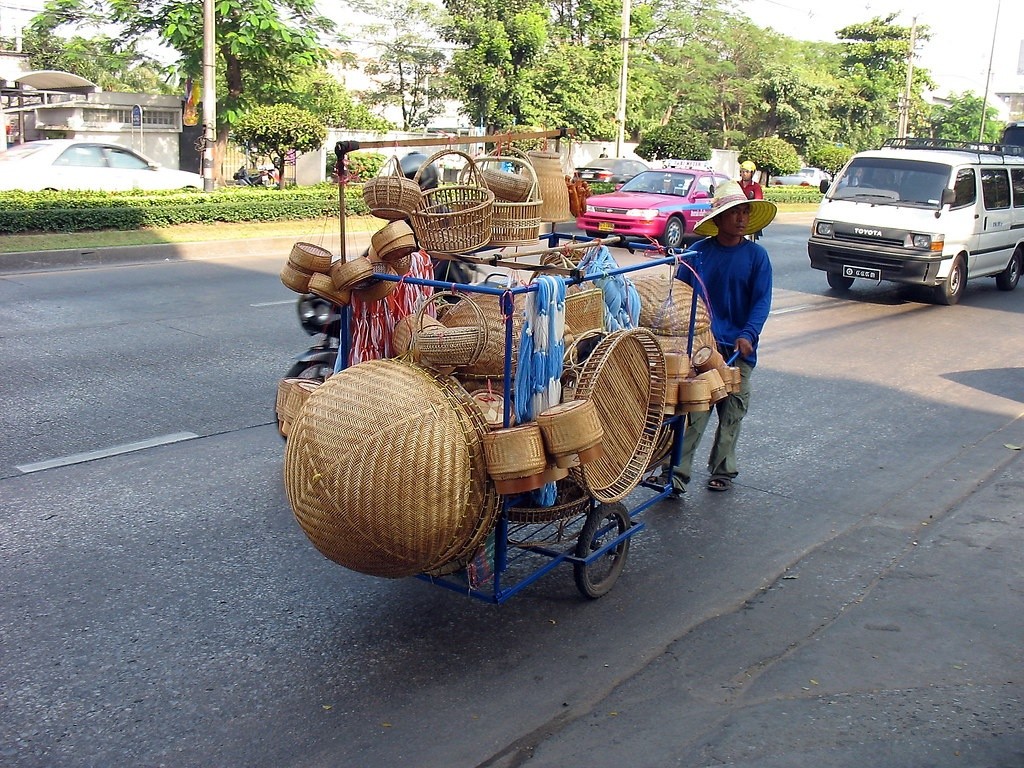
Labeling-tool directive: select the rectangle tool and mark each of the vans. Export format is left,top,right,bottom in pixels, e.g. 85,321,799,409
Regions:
808,138,1023,306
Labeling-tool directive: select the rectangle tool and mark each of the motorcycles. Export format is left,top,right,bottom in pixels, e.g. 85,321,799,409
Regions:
233,157,280,188
275,250,479,442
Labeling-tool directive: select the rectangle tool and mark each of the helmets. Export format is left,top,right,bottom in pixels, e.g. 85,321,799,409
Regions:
740,161,756,173
392,151,439,196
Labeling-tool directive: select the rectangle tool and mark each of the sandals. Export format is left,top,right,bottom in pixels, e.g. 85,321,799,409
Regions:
708,476,733,490
638,475,679,498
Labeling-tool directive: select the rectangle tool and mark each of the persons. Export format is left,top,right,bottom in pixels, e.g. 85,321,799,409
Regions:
645,192,772,494
502,149,510,172
599,147,608,158
363,151,476,304
238,165,279,187
737,161,765,241
878,172,898,192
474,147,488,188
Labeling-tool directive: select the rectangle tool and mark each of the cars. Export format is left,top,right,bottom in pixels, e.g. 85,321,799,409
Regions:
0,139,205,193
572,158,648,184
771,167,834,188
576,168,731,248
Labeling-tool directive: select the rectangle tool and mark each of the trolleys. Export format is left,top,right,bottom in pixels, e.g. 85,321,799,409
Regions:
334,126,742,606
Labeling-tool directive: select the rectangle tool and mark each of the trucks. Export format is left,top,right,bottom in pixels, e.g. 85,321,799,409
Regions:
1000,120,1024,157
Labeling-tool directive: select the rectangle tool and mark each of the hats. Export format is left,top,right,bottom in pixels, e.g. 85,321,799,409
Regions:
692,179,777,236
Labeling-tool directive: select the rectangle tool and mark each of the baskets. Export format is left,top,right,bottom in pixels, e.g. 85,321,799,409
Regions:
282,246,717,576
362,155,422,220
409,149,495,254
458,156,543,245
